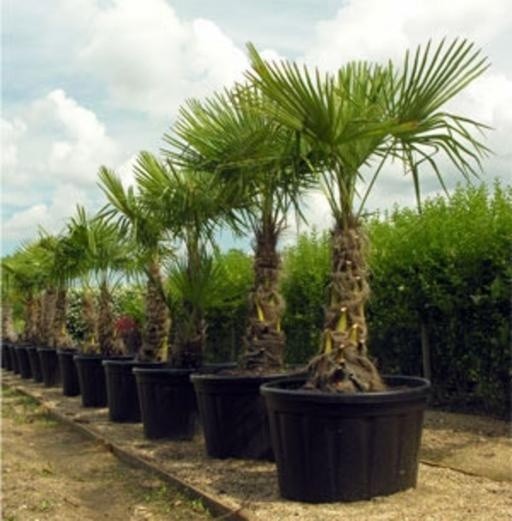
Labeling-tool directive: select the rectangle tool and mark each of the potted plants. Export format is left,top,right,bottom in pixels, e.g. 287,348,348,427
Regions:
1,202,141,425
96,35,497,505
